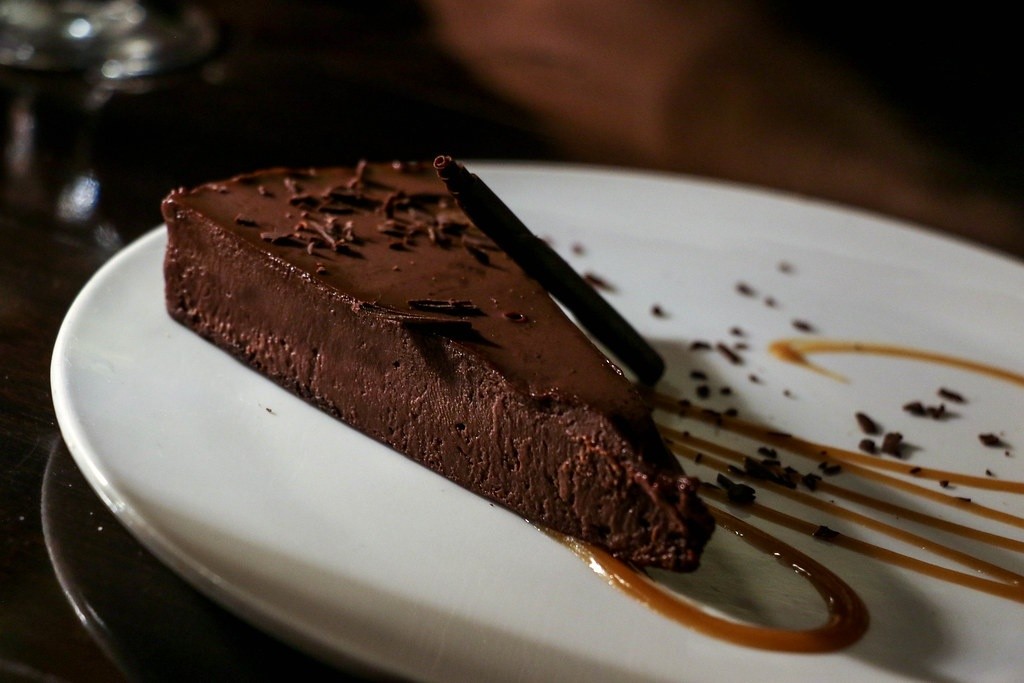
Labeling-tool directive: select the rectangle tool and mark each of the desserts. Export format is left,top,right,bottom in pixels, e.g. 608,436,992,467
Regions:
158,152,720,575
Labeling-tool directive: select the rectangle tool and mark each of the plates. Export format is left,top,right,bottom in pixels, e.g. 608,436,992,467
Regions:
50,160,1024,683
